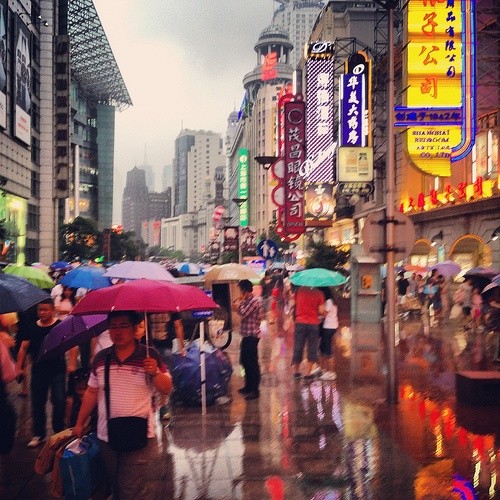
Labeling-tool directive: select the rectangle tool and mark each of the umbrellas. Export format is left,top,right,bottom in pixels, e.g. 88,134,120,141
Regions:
0,259,500,359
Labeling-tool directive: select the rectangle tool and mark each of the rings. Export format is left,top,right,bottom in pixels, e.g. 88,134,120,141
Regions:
149,366,153,369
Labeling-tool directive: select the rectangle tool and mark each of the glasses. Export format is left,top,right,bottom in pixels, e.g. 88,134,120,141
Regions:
108,324,137,331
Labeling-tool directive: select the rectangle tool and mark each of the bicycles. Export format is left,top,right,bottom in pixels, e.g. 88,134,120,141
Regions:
204,317,233,350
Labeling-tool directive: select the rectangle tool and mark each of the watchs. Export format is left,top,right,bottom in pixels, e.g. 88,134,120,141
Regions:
151,367,160,377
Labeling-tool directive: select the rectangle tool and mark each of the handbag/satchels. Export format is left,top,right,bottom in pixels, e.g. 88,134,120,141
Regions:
108,416,148,450
35,420,110,500
449,304,462,319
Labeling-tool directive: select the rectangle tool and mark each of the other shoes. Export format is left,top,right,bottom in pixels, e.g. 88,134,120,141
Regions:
28,436,44,448
18,391,27,397
311,367,327,374
302,376,314,381
294,373,301,379
238,387,248,393
319,372,336,381
245,393,257,400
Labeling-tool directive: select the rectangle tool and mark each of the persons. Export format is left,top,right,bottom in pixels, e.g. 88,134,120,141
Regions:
0,270,500,500
